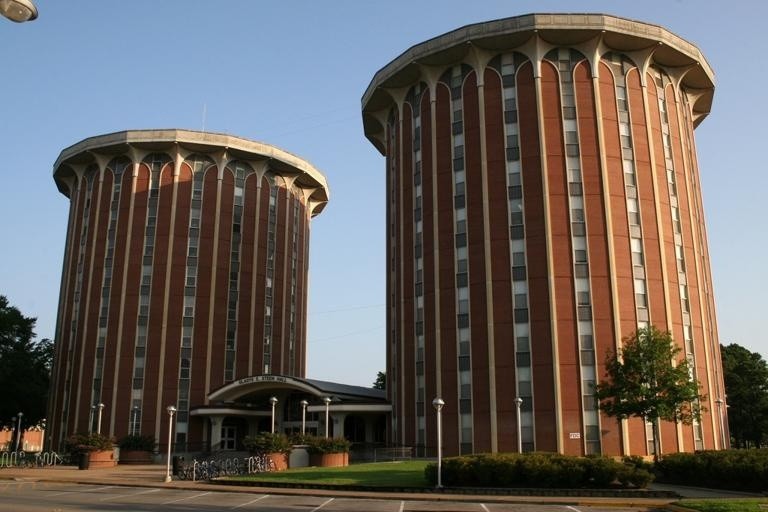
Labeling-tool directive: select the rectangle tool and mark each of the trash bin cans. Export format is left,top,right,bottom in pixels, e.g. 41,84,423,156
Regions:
172,456,184,475
78,452,89,470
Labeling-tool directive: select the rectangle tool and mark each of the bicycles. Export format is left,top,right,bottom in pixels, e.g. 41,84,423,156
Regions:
20,452,44,469
171,453,269,480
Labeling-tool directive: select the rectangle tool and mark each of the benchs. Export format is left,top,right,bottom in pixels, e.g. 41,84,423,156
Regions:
373,447,412,462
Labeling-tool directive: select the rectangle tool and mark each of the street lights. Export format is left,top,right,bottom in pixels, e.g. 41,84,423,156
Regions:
14,407,25,455
88,404,96,438
166,402,175,483
266,395,280,435
320,394,333,438
132,403,139,437
95,401,104,432
299,399,307,435
512,396,526,455
429,395,444,492
